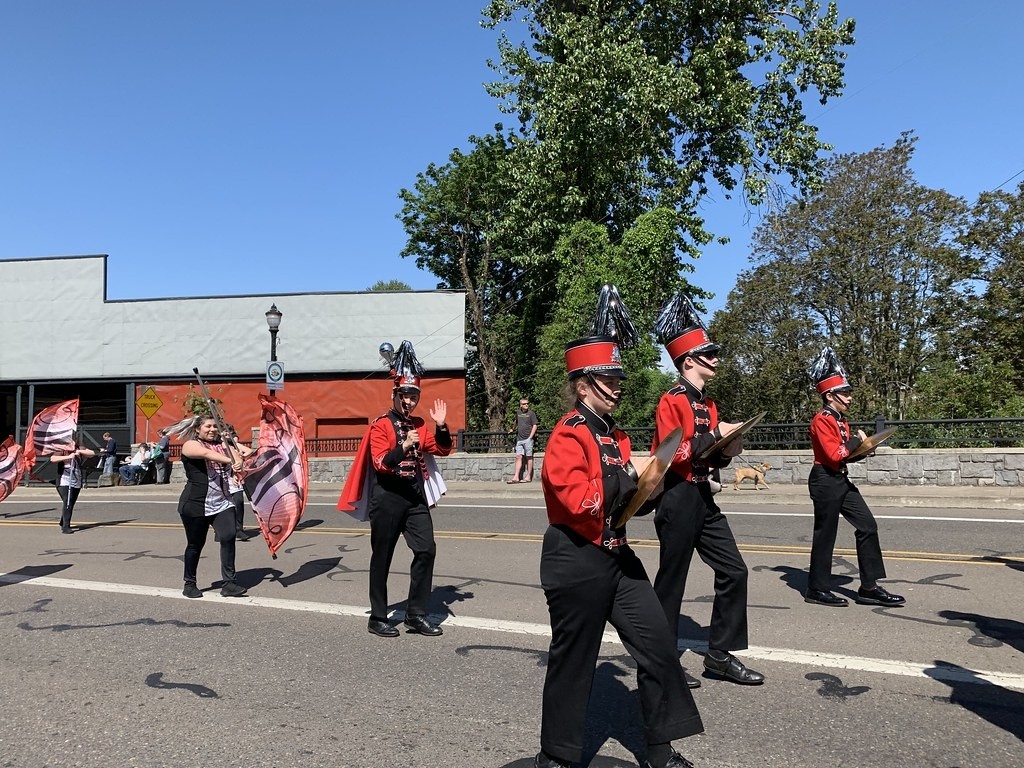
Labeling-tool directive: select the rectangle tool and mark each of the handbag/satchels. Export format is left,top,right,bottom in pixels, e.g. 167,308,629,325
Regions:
96,458,104,468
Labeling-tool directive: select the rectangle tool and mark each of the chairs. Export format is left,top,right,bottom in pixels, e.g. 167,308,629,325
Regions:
120,455,155,486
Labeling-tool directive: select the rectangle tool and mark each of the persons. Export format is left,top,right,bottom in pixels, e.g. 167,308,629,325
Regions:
49,432,96,535
119,442,151,486
804,342,907,608
99,431,117,483
334,339,455,638
648,280,767,689
162,413,250,598
150,428,171,485
536,276,706,768
506,398,540,484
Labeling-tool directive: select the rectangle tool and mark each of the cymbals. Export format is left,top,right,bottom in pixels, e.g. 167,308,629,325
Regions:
846,426,898,461
700,410,768,460
615,425,684,529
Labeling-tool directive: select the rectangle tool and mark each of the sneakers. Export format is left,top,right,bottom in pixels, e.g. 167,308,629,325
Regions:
220,583,247,597
182,583,203,598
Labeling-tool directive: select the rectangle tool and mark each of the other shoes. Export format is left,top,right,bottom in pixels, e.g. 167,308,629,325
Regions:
59,523,62,526
215,533,220,542
155,483,162,485
123,482,137,486
63,530,75,534
235,531,249,540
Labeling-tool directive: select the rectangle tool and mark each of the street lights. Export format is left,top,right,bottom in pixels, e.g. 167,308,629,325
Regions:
264,302,283,396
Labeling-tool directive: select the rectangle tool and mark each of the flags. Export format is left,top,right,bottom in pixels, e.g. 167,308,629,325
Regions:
24,396,80,472
236,391,310,558
0,434,26,502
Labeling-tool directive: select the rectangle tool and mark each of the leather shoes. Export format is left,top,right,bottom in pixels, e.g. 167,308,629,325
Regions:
857,586,906,606
404,614,443,635
534,753,571,768
644,744,694,768
685,673,701,688
367,617,399,636
805,588,849,605
703,651,764,684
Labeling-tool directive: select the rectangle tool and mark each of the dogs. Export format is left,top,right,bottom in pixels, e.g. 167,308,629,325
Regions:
731,458,773,491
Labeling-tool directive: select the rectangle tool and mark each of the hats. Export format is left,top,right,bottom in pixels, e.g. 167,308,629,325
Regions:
139,443,147,446
393,340,426,392
654,293,720,365
564,284,636,381
805,347,854,399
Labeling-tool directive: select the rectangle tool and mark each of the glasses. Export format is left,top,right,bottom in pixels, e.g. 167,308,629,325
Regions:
522,403,529,405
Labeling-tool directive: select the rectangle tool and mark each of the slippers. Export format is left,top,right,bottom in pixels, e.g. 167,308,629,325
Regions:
507,480,531,484
